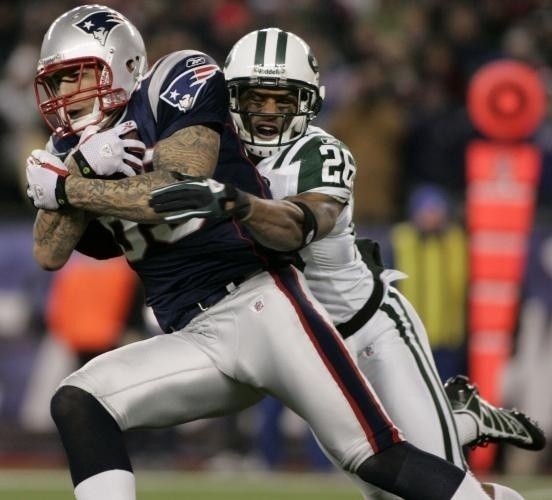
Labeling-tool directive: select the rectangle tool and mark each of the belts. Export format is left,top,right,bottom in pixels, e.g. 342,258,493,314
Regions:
337,279,384,339
172,278,245,331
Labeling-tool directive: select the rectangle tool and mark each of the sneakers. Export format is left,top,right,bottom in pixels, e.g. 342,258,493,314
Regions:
444,373,546,450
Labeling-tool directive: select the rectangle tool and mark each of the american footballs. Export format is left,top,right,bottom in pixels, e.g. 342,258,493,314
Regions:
95,127,145,179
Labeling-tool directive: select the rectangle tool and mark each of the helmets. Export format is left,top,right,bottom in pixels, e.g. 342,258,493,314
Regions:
221,28,324,158
34,3,149,141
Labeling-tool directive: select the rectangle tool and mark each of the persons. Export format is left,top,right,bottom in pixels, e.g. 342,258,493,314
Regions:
2,2,551,478
74,27,549,499
23,2,492,500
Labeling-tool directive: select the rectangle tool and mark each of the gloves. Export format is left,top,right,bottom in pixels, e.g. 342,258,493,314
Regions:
148,170,250,230
71,119,147,178
25,149,73,211
